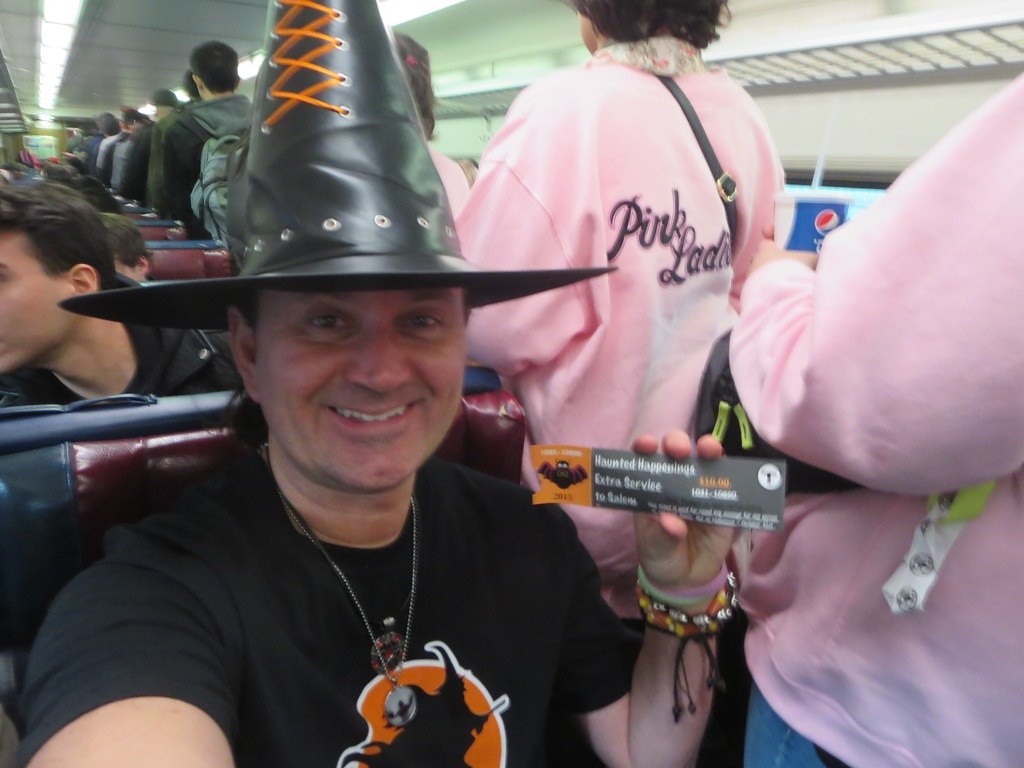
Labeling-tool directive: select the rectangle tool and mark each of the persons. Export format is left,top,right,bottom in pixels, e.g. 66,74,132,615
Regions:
0,40,254,410
391,31,500,392
729,69,1024,768
450,0,793,618
21,281,742,768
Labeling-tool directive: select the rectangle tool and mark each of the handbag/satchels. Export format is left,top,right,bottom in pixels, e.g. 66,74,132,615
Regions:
692,326,864,493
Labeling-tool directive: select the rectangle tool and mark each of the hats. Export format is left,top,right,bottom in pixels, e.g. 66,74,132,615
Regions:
59,0,620,331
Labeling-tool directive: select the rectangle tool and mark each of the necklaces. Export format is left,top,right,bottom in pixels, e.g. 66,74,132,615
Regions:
276,490,418,727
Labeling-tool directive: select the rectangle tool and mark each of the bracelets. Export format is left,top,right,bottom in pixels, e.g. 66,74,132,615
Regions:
635,565,738,722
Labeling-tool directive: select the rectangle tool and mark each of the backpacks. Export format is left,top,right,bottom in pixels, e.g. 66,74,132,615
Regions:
175,110,253,250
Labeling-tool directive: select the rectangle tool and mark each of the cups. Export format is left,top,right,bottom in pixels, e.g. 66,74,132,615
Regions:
773,191,853,253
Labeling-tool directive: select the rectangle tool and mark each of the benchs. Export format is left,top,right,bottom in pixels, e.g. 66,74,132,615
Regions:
1,145,529,743
